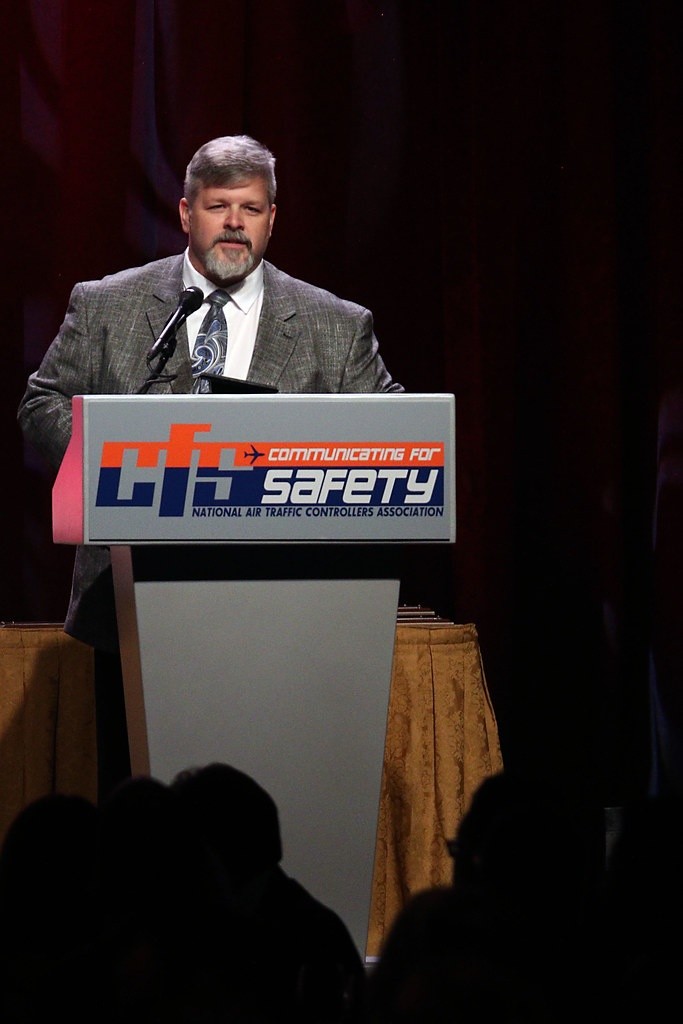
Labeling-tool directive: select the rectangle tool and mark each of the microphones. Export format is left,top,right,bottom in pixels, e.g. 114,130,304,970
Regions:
146,286,204,363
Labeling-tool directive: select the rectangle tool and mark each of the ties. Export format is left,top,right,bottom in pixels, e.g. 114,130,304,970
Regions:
190,289,231,394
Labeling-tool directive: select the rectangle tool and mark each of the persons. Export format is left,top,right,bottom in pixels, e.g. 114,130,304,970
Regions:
18,137,406,795
0,755,683,1024
